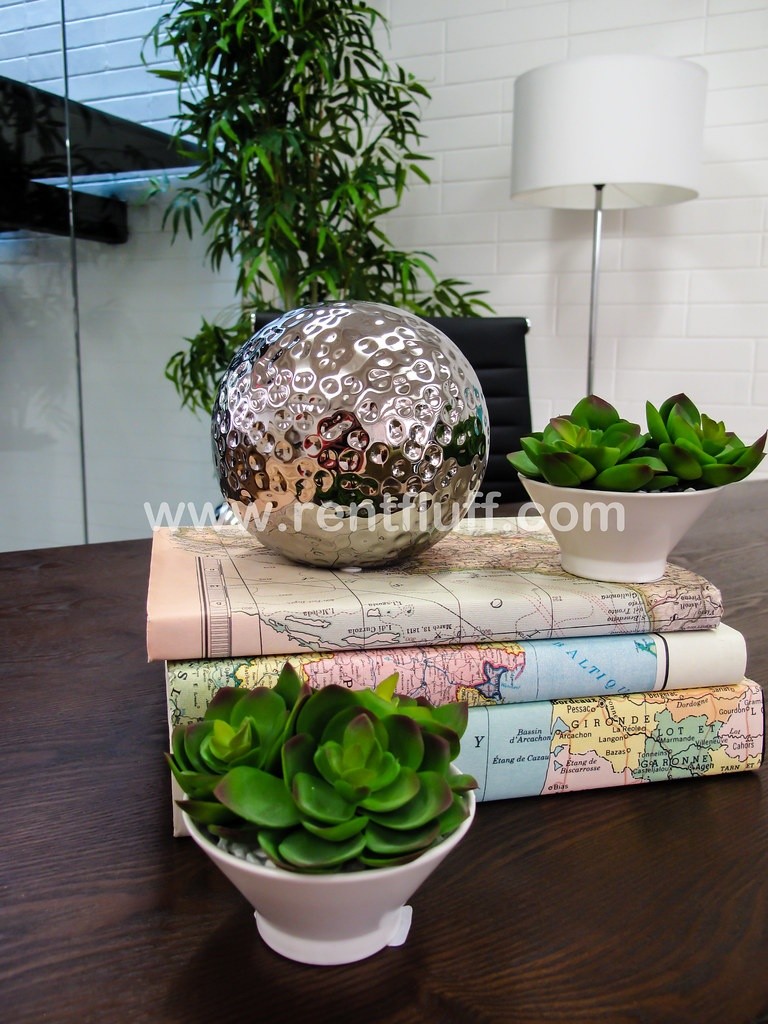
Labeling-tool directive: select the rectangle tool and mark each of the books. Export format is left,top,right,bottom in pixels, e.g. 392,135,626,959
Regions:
144,512,764,837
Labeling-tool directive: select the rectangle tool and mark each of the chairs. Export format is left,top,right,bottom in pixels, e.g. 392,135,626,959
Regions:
250,313,533,516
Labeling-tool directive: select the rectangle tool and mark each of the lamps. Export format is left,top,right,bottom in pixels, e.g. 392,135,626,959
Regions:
510,56,700,395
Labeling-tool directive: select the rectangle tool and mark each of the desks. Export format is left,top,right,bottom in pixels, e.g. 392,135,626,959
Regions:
0,478,767,1024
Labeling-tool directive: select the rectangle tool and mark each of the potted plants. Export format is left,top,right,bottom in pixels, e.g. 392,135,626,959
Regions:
164,657,479,966
506,393,768,583
